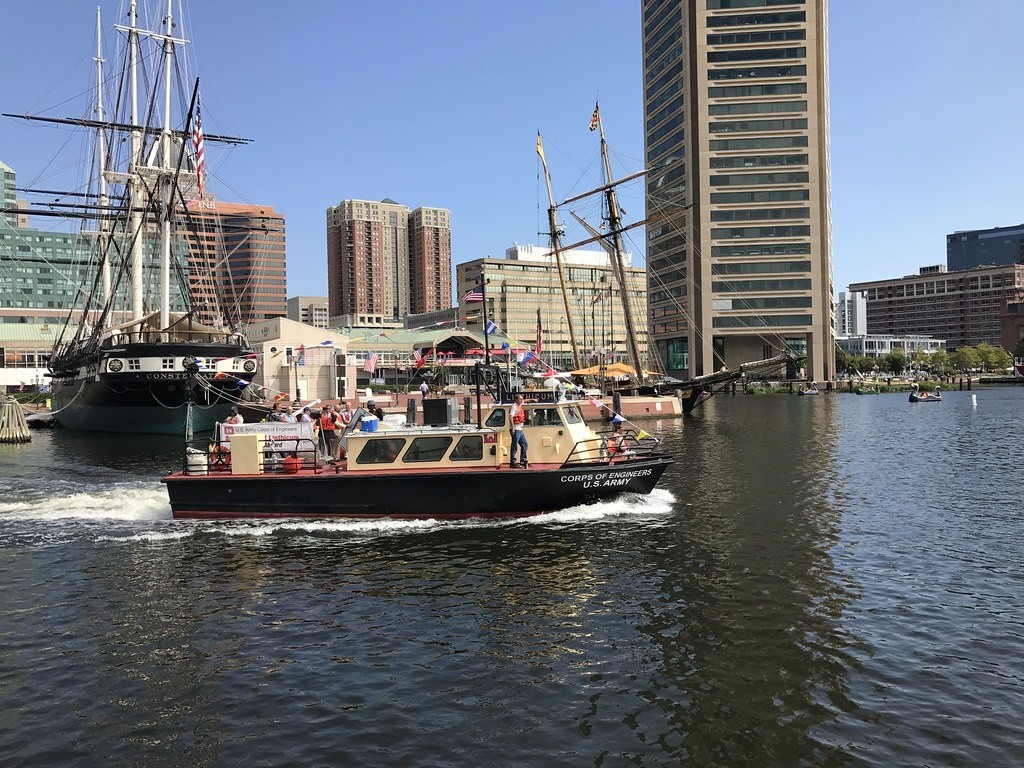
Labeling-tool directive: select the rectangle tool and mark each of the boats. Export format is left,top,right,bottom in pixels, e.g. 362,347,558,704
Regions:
908,383,943,402
798,380,819,395
239,377,685,419
26,410,55,428
856,381,881,396
160,262,675,524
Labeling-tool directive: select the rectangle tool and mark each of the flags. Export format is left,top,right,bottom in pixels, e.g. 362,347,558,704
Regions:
274,392,289,402
363,351,378,373
536,308,544,355
192,91,206,200
604,285,613,298
257,385,269,391
237,378,252,387
214,372,228,380
482,321,497,336
414,347,421,361
536,129,551,181
590,292,602,307
461,284,485,302
587,346,602,362
603,346,616,360
589,100,599,131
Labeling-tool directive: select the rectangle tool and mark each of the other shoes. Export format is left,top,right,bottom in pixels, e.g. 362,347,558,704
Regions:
510,465,519,468
520,463,532,467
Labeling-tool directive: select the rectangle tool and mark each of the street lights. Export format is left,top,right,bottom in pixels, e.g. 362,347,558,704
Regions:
392,349,400,405
982,362,985,373
335,348,343,404
291,355,301,405
954,364,957,378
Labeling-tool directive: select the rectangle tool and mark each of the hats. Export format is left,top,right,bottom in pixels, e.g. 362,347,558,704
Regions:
229,406,238,411
304,407,311,412
367,400,376,405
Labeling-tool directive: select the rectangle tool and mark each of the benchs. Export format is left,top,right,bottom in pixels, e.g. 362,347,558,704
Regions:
470,388,497,395
577,389,603,399
441,391,455,398
359,396,394,408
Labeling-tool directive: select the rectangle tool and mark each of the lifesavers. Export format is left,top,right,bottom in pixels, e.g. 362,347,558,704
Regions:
211,446,231,467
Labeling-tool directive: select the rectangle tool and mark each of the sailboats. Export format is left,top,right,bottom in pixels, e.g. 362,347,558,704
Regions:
42,0,261,439
493,101,807,415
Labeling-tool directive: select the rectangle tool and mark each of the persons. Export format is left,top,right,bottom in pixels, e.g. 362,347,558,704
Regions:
267,402,355,459
224,406,244,424
509,394,532,469
609,425,630,453
419,381,429,404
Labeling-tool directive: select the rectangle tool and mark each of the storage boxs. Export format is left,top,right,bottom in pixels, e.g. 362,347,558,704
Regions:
361,416,378,431
